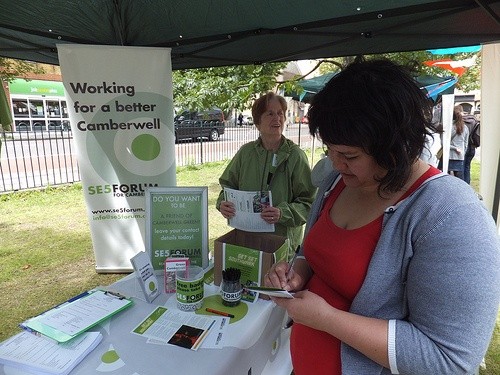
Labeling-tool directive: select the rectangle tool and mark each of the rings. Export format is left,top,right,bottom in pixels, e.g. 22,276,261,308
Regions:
271,217,274,221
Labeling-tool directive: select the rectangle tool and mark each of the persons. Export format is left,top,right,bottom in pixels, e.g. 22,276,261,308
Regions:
264,53,500,375
216,92,319,263
441,110,469,180
239,113,243,127
454,105,476,185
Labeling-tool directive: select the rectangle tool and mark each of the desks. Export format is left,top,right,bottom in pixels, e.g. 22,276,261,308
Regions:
0,257,293,375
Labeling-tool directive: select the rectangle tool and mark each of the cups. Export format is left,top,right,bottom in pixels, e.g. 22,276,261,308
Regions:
175,265,204,312
220,277,242,307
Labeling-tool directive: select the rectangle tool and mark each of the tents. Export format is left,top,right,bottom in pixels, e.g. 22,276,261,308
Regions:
284,67,456,105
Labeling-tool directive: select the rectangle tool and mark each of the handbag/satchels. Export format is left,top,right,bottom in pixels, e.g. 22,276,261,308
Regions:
436,147,443,159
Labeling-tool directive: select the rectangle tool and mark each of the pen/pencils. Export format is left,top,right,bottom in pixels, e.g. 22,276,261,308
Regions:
285,245,300,281
222,267,241,281
206,307,234,318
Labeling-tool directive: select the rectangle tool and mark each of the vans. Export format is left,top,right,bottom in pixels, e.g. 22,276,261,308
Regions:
174,108,225,141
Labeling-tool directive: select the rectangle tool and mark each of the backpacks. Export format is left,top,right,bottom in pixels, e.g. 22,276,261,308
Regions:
465,117,480,150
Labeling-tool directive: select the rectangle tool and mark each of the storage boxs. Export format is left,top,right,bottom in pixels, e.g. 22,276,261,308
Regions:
214,228,289,287
